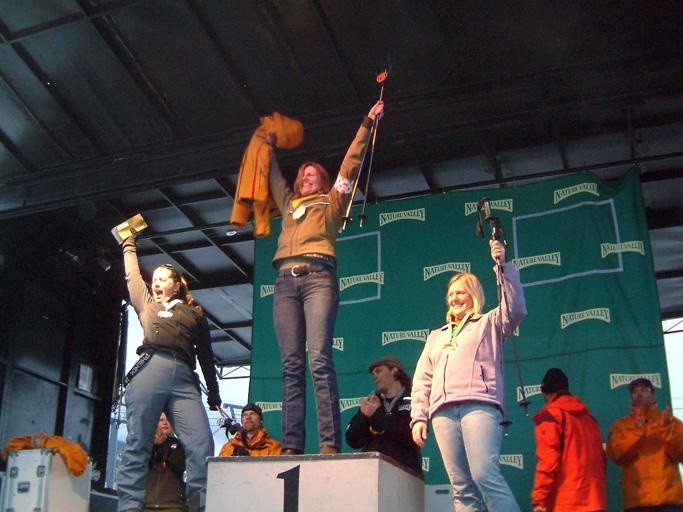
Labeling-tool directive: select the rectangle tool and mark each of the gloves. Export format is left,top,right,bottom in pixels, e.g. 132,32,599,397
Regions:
122,227,138,249
207,389,222,411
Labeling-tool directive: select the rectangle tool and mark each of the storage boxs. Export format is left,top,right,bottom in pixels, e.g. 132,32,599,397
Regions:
4,448,92,511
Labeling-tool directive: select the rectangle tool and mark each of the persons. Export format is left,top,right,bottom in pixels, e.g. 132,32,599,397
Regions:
218,403,281,455
258,101,387,455
408,241,528,511
604,377,683,511
529,367,609,511
142,408,187,511
110,226,224,511
344,356,422,474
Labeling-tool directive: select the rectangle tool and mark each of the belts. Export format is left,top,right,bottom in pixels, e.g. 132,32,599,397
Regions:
278,262,325,276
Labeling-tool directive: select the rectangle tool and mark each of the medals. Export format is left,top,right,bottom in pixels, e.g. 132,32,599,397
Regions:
291,205,305,220
441,339,457,353
156,310,173,318
369,425,384,435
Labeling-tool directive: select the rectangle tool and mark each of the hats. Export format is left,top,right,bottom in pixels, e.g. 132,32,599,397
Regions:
629,378,653,392
242,403,262,417
369,356,401,373
542,368,568,387
